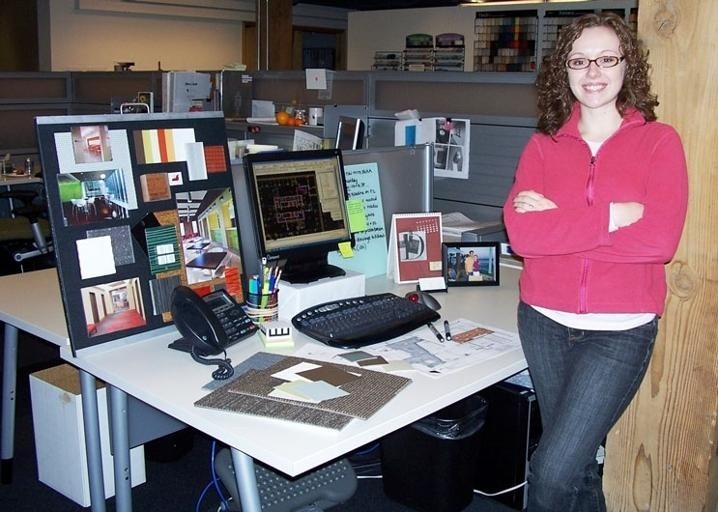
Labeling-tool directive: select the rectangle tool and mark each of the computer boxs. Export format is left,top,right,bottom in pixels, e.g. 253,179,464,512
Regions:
487,382,541,511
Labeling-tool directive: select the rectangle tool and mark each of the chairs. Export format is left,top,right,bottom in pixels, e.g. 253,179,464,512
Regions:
213,439,355,512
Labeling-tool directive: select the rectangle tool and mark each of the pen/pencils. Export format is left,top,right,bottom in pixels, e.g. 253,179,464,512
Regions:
427,321,444,342
444,320,451,340
249,257,282,325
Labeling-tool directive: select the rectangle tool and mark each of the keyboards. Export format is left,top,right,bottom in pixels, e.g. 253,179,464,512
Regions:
292,292,439,348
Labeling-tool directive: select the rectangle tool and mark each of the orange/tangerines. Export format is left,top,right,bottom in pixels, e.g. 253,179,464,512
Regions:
295,119,305,127
288,118,295,126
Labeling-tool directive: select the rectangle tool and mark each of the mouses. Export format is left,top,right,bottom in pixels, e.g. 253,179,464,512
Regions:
407,289,442,313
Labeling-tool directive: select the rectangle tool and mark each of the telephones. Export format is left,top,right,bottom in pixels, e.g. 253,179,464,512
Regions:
170,285,257,380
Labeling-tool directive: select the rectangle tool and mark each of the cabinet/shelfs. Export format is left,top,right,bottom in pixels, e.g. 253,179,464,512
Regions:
460,1,639,72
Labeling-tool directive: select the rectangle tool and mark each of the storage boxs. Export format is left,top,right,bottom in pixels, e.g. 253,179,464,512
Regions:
29,363,146,507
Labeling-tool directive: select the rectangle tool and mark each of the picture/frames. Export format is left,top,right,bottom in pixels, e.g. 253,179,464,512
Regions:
442,241,500,287
34,110,249,358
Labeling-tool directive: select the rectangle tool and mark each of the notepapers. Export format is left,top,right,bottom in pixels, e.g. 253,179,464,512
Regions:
346,199,367,233
338,241,354,258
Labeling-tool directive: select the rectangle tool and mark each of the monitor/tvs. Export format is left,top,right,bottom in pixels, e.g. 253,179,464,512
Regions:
243,149,351,285
335,116,365,149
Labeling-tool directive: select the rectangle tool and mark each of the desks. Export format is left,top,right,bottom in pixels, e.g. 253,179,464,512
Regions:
0,268,69,460
60,255,529,512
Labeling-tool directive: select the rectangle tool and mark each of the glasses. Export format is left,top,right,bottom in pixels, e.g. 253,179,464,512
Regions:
567,56,626,69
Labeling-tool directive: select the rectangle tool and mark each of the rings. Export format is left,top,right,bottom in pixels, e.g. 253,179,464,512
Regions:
529,205,534,208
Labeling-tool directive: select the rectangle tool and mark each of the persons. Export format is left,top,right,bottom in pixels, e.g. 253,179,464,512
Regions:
501,12,689,512
472,255,480,281
464,250,473,281
